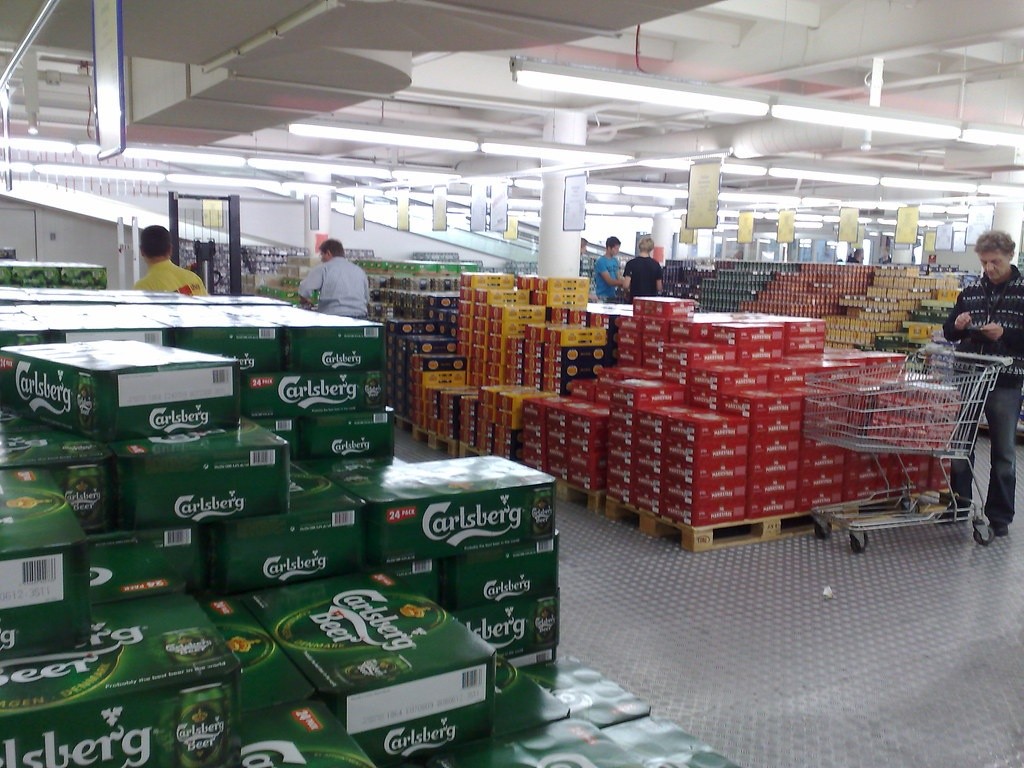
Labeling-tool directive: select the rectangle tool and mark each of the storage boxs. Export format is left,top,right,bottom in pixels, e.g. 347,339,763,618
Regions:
1,246,980,768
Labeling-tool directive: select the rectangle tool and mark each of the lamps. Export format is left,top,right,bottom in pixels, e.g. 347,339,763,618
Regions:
1,54,1022,240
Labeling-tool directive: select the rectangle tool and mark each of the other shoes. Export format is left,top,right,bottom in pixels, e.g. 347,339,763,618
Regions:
988,521,1008,537
935,510,969,524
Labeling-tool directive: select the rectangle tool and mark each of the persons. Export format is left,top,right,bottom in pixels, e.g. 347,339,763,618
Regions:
297,239,371,321
594,236,663,305
579,238,598,303
939,230,1024,536
133,225,208,297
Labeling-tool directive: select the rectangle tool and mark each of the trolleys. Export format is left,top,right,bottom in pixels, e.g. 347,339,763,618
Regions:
801,340,1014,553
